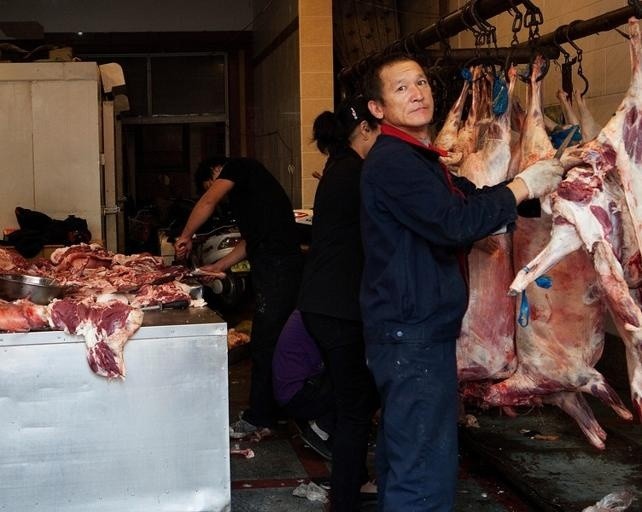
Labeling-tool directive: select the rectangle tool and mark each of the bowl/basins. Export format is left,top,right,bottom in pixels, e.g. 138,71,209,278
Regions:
0,271,66,306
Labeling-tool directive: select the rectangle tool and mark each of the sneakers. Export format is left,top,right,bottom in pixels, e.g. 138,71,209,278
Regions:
230,418,258,439
299,424,333,460
360,478,378,494
295,420,312,433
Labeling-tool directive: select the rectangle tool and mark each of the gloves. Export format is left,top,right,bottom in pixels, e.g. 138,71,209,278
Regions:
513,157,565,201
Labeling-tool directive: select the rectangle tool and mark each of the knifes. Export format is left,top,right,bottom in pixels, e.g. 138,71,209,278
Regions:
112,273,177,296
553,125,578,162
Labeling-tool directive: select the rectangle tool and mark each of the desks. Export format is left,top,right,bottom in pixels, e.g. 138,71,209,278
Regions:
0,295,231,512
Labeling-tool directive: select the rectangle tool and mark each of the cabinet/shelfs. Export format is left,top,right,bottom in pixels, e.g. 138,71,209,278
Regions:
0,57,231,254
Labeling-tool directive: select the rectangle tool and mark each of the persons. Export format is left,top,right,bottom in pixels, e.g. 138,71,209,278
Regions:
351,43,566,511
296,96,501,511
269,302,338,462
173,149,305,443
